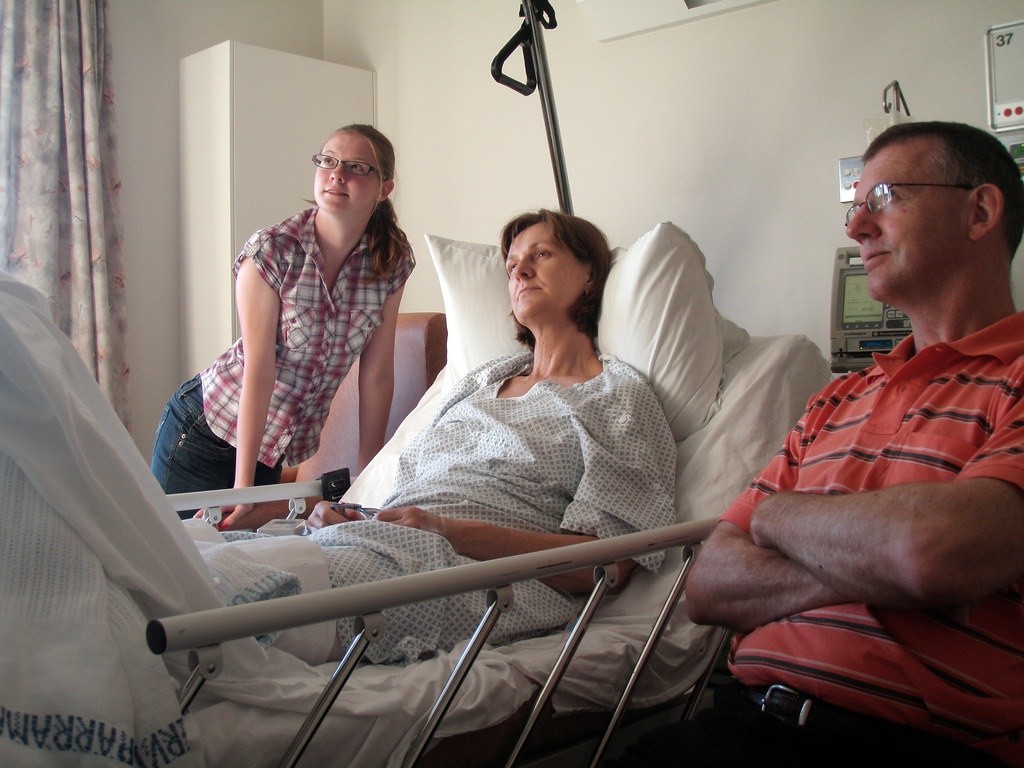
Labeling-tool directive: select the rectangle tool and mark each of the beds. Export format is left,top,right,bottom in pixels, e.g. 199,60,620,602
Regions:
0,273,839,768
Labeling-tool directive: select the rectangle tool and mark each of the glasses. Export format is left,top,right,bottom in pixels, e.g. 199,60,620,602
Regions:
330,502,381,520
312,154,378,176
845,182,973,228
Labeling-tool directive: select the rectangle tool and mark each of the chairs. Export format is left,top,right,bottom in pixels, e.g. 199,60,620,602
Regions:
208,312,447,528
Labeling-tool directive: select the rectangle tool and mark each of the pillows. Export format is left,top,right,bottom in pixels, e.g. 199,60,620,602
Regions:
423,221,722,440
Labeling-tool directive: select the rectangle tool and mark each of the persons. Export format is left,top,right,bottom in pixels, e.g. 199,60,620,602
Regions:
212,209,678,665
684,121,1024,768
149,123,418,536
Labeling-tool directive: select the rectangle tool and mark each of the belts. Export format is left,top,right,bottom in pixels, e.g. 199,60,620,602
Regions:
729,674,959,768
